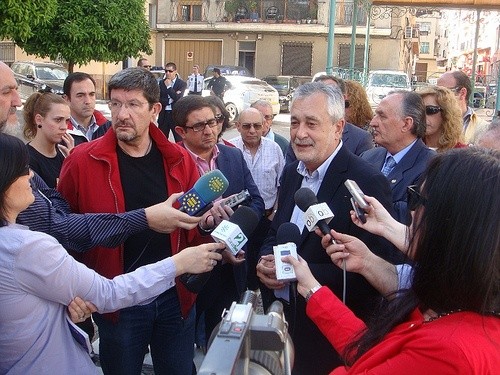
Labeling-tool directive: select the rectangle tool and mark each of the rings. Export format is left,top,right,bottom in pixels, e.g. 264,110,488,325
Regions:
210,258,214,265
79,316,84,319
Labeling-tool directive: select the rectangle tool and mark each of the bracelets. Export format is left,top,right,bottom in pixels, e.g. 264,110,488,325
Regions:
272,209,277,212
305,284,322,301
205,215,213,224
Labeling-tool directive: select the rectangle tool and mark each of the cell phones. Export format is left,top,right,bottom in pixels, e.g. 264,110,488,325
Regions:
350,197,367,224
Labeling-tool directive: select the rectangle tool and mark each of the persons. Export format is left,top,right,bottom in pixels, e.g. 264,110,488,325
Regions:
356,88,441,225
187,65,204,96
138,59,150,70
207,67,232,104
157,63,187,144
248,99,290,158
0,62,500,375
221,108,287,221
60,72,111,149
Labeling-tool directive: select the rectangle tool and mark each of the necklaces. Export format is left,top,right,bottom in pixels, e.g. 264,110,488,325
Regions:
424,307,463,322
120,139,152,156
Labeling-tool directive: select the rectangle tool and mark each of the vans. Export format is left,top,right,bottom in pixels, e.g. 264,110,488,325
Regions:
205,65,252,83
312,71,412,117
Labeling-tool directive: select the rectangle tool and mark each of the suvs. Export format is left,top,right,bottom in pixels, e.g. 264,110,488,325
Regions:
258,76,313,113
10,61,69,101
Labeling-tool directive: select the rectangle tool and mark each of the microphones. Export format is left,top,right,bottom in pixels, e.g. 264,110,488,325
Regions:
178,169,228,218
294,187,336,241
184,204,259,293
276,220,299,298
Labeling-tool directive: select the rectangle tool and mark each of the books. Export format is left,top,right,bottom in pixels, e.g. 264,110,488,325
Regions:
65,312,92,354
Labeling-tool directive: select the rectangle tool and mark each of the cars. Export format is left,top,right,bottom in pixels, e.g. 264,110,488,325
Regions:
183,75,280,128
151,68,181,85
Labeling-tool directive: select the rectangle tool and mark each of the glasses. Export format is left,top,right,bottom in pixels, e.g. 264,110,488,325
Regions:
406,185,427,211
165,69,174,73
426,106,441,115
264,115,274,120
16,166,31,175
142,64,152,68
240,124,264,130
216,114,225,120
346,98,351,110
182,118,222,131
108,99,152,110
